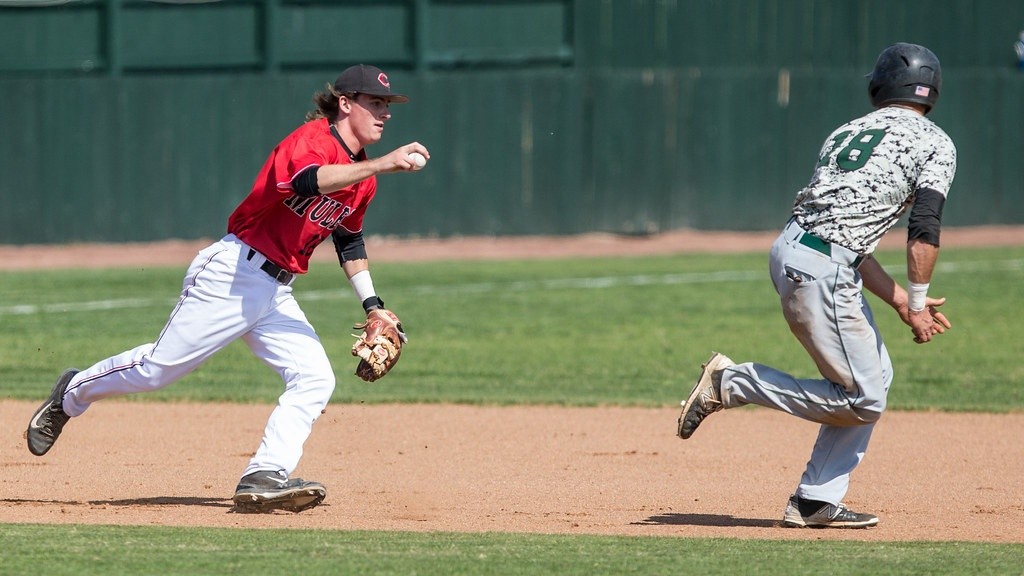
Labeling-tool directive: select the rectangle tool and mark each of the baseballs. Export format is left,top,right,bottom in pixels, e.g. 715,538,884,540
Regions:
408,152,426,170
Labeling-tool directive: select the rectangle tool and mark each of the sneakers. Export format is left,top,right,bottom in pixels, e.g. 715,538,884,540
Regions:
783,494,879,528
678,350,735,439
233,469,326,514
27,368,79,456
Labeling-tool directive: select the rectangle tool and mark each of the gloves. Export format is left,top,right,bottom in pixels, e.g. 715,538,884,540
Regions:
785,272,803,282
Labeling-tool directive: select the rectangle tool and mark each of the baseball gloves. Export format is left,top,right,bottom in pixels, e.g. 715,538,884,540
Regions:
351,309,408,381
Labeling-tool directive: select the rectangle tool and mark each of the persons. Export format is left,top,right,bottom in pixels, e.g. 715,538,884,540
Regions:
677,44,959,529
28,64,431,511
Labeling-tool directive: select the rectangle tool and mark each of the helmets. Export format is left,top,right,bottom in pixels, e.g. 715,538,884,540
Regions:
868,42,941,115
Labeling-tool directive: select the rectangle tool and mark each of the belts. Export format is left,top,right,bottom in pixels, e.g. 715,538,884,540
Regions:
785,218,864,269
246,249,296,286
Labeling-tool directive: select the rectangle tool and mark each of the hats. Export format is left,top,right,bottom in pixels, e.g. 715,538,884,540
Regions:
335,64,409,103
